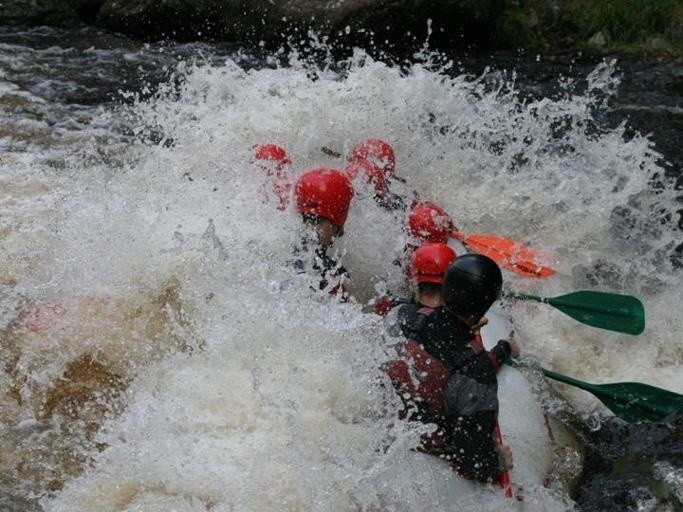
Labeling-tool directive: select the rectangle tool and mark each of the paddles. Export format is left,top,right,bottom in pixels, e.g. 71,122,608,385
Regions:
449,229,556,278
504,358,683,422
498,290,646,334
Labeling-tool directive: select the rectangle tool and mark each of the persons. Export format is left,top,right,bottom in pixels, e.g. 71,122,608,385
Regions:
249,138,454,315
375,242,521,484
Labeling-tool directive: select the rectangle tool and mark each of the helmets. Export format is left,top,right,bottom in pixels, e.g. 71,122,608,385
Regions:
346,162,386,197
295,170,353,228
347,138,395,177
255,145,289,175
444,254,502,320
252,181,290,211
410,242,454,286
407,205,453,244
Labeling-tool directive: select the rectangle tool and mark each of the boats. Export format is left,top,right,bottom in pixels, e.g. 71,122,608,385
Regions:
318,159,573,512
